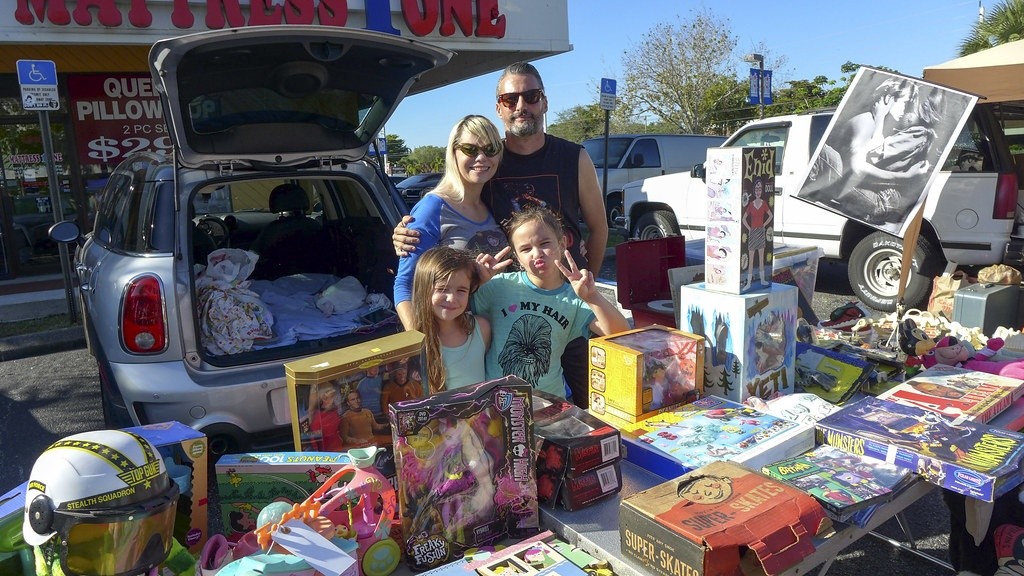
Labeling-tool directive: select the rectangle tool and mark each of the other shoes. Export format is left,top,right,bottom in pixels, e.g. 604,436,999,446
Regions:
455,525,466,544
444,527,454,543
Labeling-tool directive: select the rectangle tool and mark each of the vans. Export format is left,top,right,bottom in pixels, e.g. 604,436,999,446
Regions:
578,136,749,230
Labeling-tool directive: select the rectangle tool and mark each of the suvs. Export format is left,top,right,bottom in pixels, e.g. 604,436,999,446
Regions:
54,24,459,454
620,109,1016,314
395,173,442,211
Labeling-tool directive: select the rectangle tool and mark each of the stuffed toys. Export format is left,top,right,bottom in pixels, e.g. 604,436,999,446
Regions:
897,318,1023,383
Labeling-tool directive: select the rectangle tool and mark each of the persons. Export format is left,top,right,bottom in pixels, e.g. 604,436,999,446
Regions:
406,245,493,397
305,341,673,548
392,63,610,409
394,114,512,331
798,75,949,223
89,185,105,211
469,198,633,412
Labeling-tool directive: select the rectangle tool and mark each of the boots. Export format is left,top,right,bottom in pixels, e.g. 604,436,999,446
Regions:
759,271,769,285
742,273,752,291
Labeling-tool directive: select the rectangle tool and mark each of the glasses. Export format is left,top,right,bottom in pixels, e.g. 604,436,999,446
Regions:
497,88,546,108
454,142,501,158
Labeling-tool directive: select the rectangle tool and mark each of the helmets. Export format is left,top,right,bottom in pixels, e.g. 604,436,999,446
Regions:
22,428,181,576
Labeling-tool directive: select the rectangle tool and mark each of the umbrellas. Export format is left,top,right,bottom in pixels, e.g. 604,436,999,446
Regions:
925,37,1024,104
